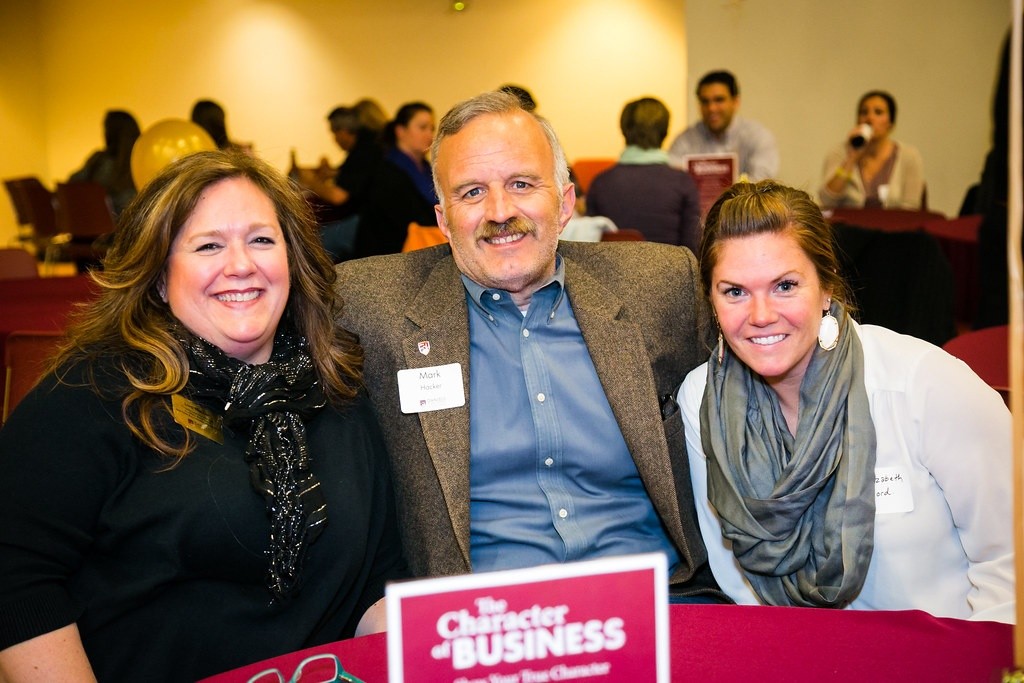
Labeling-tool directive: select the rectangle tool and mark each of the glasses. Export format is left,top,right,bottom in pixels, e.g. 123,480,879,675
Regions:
248,654,367,683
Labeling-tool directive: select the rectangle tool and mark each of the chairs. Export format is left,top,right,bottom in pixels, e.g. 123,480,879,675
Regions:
0,249,40,279
5,177,114,277
2,329,69,426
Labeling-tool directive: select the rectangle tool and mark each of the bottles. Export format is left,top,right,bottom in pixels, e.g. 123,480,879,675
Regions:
851,121,872,149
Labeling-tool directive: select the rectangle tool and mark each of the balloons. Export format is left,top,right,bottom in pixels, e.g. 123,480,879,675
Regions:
129,118,220,194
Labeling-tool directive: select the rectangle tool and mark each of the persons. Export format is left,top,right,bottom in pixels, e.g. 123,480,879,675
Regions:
0,152,412,683
582,97,702,259
282,97,440,264
959,23,1011,328
668,68,778,180
496,84,617,243
816,89,925,210
676,182,1016,624
336,91,733,605
61,97,252,263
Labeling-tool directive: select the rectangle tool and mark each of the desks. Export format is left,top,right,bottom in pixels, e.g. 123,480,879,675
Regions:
196,604,1015,683
821,211,983,310
0,277,110,335
